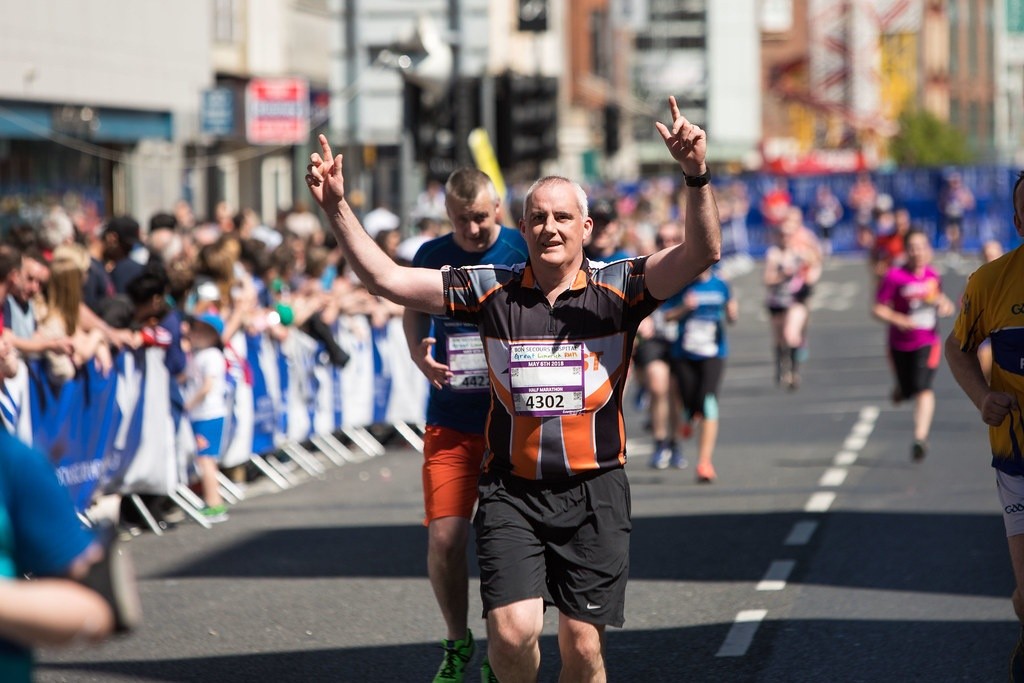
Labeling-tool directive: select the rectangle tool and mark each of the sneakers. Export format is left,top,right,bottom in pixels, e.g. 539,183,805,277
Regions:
432,627,499,683
694,462,717,483
197,503,228,523
649,446,688,469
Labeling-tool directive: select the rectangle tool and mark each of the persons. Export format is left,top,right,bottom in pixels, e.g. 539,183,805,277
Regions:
941,169,1024,683
176,304,231,524
578,158,1005,485
0,423,142,681
0,172,456,379
298,89,727,681
396,165,528,682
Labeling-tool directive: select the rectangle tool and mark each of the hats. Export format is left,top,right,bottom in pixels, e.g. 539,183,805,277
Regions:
185,311,225,351
